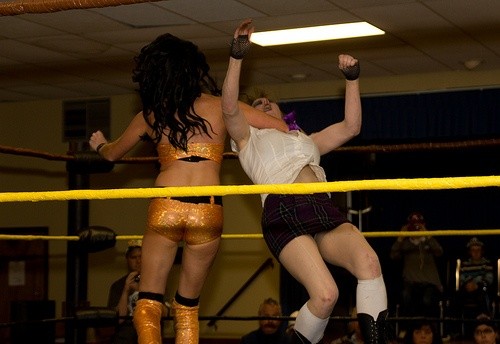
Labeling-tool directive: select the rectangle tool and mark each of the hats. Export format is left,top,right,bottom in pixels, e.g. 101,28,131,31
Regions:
408,212,424,226
467,237,483,247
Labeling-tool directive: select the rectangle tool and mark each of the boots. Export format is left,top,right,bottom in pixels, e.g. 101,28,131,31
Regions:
285,327,324,344
357,308,391,344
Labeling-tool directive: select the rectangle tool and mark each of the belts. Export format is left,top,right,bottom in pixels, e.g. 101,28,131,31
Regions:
171,196,222,205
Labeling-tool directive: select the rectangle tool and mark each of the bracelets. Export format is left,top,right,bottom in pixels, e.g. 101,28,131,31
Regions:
97,142,106,153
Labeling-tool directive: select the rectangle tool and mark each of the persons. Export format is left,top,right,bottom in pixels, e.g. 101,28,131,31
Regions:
405,318,442,343
110,245,144,324
472,318,499,344
221,19,387,344
89,33,290,344
243,298,290,344
390,212,445,337
330,308,364,344
460,236,495,318
